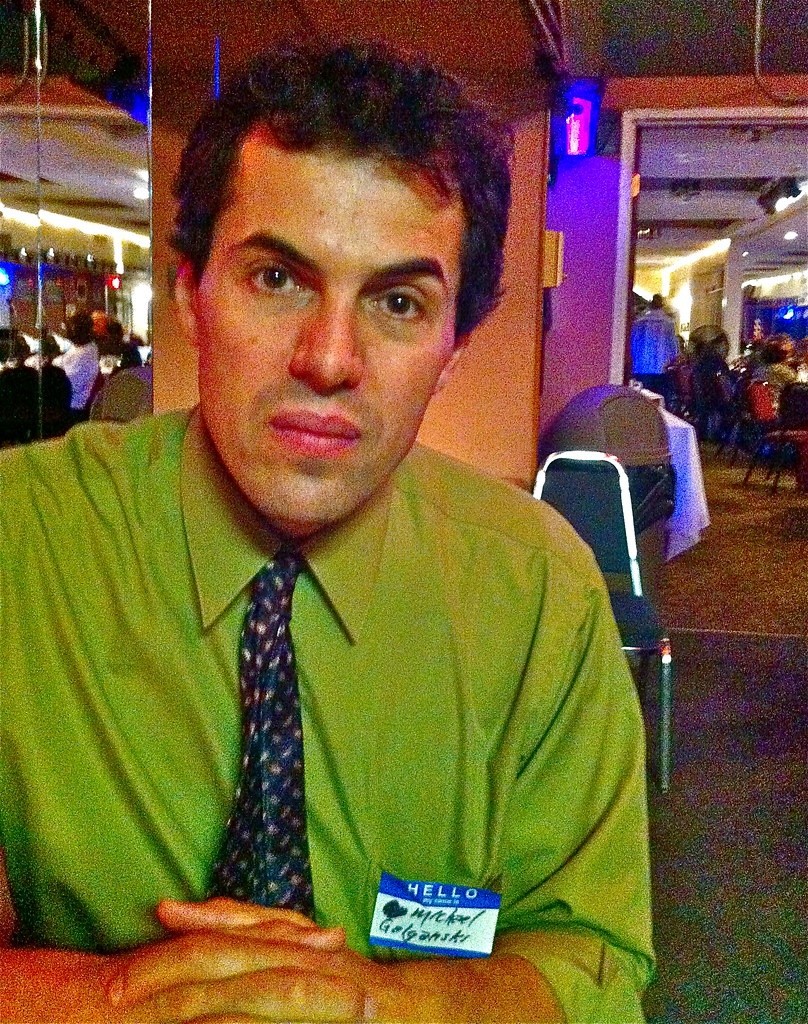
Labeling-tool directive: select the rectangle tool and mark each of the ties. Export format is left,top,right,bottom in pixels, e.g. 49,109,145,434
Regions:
205,550,315,920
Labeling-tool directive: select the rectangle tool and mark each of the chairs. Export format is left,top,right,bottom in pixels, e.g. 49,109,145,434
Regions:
700,369,748,458
532,450,675,797
770,381,808,495
736,378,779,487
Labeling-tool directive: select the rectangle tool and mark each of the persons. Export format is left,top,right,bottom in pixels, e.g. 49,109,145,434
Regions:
2,42,656,1024
678,334,808,473
1,312,144,445
636,296,673,408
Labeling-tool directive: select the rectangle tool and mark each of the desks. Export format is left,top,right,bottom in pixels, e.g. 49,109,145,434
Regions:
660,406,712,566
